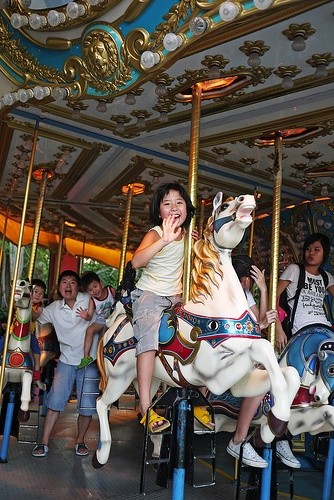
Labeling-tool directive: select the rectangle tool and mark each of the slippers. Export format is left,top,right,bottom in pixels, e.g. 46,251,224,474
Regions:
76,443,89,456
31,444,49,456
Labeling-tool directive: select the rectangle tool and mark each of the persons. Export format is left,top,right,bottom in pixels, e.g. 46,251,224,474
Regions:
32,270,100,456
1,279,45,381
75,271,118,369
131,182,218,434
227,255,302,468
270,234,334,353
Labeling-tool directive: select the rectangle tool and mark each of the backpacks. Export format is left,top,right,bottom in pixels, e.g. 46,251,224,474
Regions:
279,263,329,327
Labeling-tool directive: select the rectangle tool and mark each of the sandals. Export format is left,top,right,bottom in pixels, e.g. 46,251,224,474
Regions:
140,408,172,433
77,356,94,368
193,405,215,430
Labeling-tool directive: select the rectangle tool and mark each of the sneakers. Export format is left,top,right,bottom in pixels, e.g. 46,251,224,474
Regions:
275,439,301,469
225,437,269,468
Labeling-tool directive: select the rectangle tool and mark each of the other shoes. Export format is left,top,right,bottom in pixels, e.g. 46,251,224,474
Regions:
33,370,40,381
